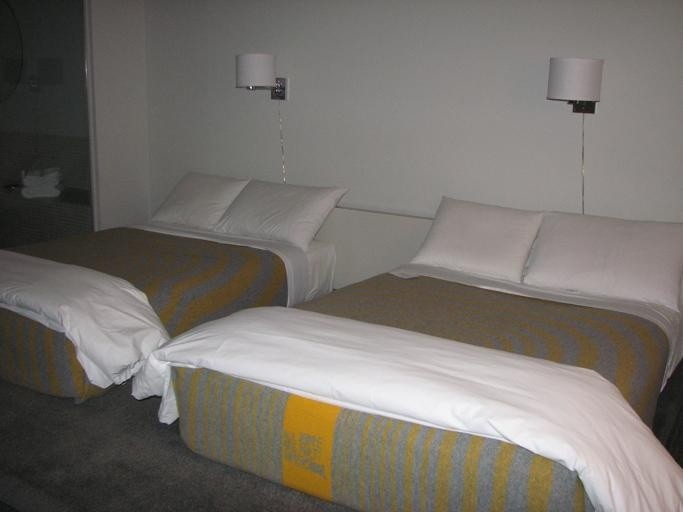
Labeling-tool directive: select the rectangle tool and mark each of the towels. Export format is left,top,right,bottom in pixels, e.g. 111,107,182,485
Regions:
20,167,65,200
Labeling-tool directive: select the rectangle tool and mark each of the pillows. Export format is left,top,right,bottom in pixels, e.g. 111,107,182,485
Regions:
211,179,349,251
145,171,253,232
409,196,543,284
523,210,683,314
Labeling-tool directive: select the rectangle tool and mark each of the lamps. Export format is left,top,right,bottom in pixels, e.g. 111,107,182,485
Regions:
546,57,604,216
236,53,287,184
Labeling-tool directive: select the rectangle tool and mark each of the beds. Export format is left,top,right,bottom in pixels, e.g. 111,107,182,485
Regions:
131,263,683,512
0,223,337,406
